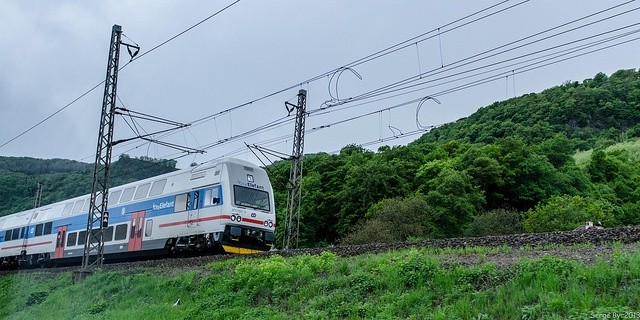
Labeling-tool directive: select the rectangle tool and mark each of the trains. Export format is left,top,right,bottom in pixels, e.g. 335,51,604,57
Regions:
0,158,277,271
